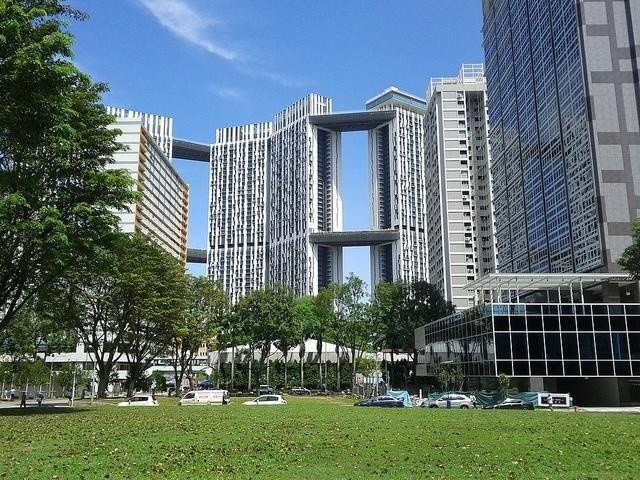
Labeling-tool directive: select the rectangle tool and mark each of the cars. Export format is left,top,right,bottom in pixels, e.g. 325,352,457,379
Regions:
420,392,474,408
483,397,534,408
354,396,404,408
242,393,287,405
118,395,160,407
197,380,214,388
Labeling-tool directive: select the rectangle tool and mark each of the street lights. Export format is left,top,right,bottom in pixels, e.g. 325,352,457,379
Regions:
88,345,97,404
376,345,382,396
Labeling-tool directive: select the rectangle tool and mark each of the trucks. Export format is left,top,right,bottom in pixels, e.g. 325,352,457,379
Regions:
176,389,231,406
252,384,274,393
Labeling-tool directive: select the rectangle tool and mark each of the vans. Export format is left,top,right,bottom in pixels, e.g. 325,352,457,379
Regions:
291,387,310,395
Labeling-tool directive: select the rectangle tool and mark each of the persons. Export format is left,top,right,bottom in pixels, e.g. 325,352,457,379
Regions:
67,388,72,406
20,392,27,407
38,392,44,406
10,387,16,400
4,388,8,399
471,392,477,409
547,394,554,412
